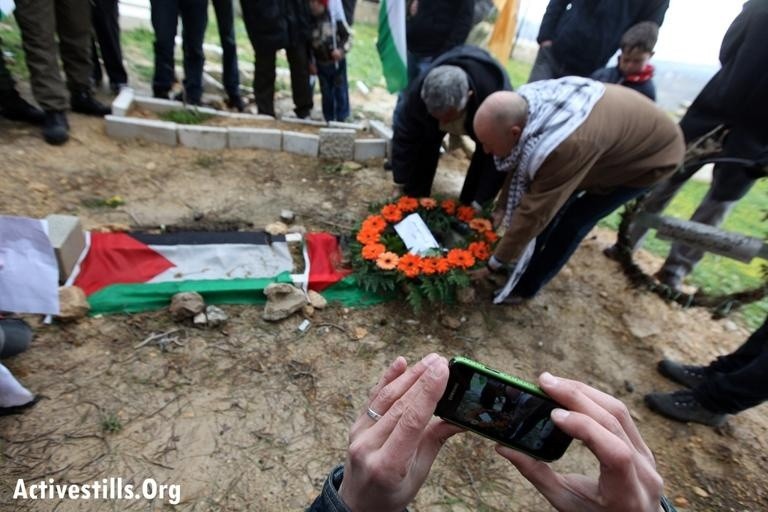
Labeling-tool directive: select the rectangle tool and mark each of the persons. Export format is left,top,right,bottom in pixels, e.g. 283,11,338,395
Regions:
645,317,768,426
604,0,768,287
467,77,686,305
391,45,516,207
303,353,678,512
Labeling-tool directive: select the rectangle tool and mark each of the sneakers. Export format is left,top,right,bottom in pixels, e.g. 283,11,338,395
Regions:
600,243,631,263
655,354,713,387
642,387,727,429
653,267,682,294
492,285,525,305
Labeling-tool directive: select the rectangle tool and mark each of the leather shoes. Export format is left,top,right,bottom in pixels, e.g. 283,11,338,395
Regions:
40,110,73,147
70,92,114,114
0,95,46,125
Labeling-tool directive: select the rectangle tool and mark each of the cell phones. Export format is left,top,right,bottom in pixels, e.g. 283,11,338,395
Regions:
433,356,575,463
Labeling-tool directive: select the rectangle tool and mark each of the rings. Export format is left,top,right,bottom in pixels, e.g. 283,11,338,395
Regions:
367,404,382,422
